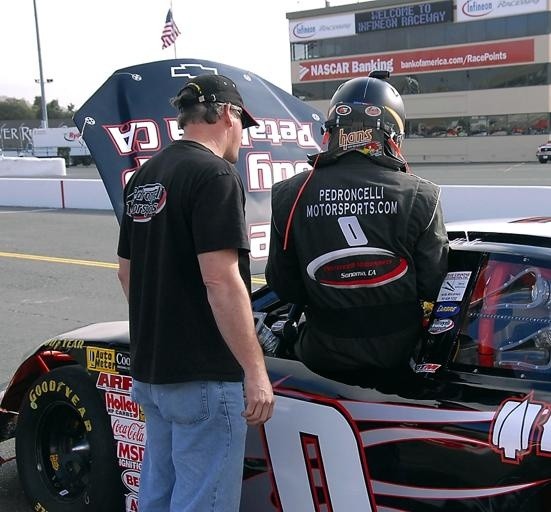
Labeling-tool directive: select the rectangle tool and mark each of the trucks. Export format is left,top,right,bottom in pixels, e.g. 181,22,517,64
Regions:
19,126,93,167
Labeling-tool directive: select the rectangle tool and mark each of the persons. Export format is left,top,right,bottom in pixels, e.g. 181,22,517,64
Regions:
264,69,450,397
117,73,274,512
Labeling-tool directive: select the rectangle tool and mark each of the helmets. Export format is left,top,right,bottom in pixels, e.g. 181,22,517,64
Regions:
322,74,408,156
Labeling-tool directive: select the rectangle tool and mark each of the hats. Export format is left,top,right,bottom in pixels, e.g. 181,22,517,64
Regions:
174,73,262,130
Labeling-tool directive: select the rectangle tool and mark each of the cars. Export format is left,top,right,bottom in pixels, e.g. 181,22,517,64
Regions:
537,141,551,163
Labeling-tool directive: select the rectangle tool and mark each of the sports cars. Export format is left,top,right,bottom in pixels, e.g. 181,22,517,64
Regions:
0,59,551,512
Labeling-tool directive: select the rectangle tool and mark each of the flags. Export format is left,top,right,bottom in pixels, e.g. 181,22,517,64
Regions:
160,8,181,51
